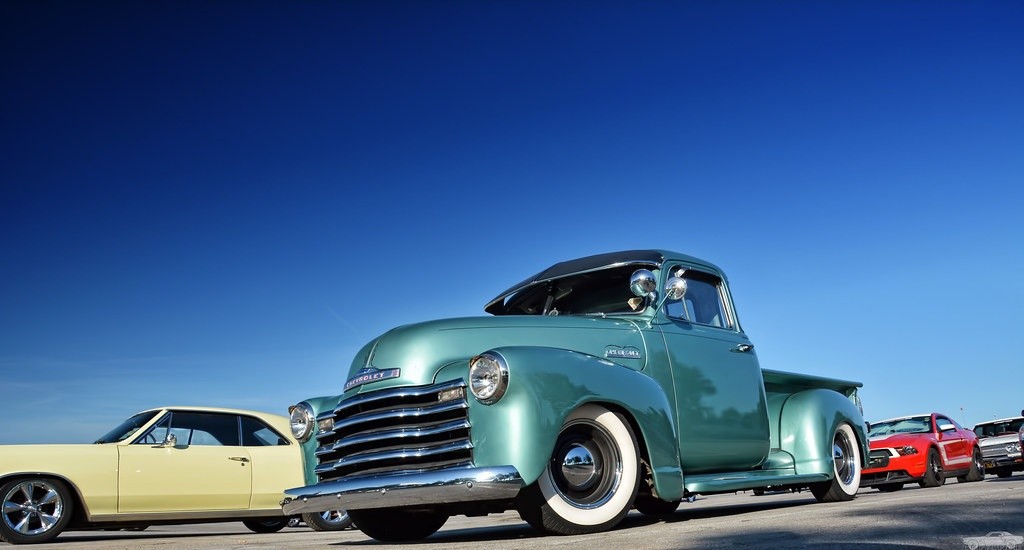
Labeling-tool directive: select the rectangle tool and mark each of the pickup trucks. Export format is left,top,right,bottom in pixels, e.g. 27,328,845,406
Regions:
281,249,870,536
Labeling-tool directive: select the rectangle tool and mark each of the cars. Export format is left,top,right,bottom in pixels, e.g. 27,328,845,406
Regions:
0,406,303,545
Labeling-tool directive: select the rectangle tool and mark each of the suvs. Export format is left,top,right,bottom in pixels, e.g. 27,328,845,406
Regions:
857,414,985,490
972,416,1024,478
